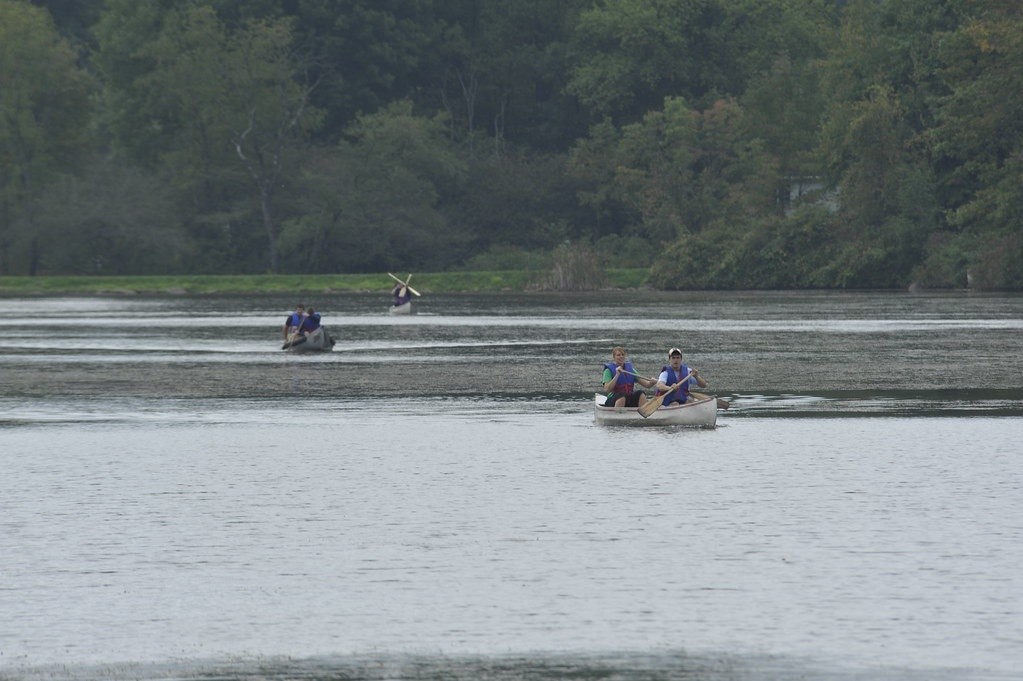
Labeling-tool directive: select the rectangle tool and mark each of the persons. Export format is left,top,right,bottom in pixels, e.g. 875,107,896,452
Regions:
282,304,306,341
656,347,706,407
298,307,321,336
601,347,657,407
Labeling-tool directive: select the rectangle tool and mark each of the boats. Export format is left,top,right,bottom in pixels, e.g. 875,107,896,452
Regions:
282,325,335,351
594,392,718,428
389,302,418,315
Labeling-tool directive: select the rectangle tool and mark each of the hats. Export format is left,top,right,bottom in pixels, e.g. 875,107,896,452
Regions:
668,347,681,356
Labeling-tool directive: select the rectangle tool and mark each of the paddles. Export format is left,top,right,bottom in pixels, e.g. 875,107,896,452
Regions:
619,367,730,410
388,272,422,297
636,367,697,419
398,274,412,297
287,315,306,352
